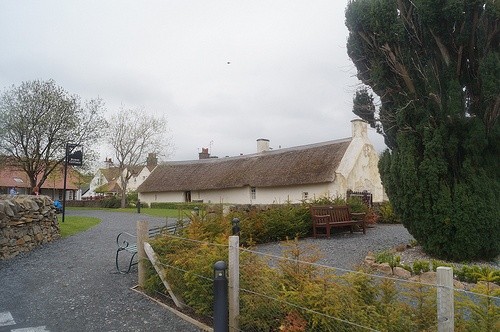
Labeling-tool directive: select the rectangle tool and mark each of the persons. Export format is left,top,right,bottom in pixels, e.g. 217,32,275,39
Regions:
10,186,19,195
30,186,40,195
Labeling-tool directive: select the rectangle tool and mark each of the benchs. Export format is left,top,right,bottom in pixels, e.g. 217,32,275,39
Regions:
310,202,366,240
115,217,196,275
